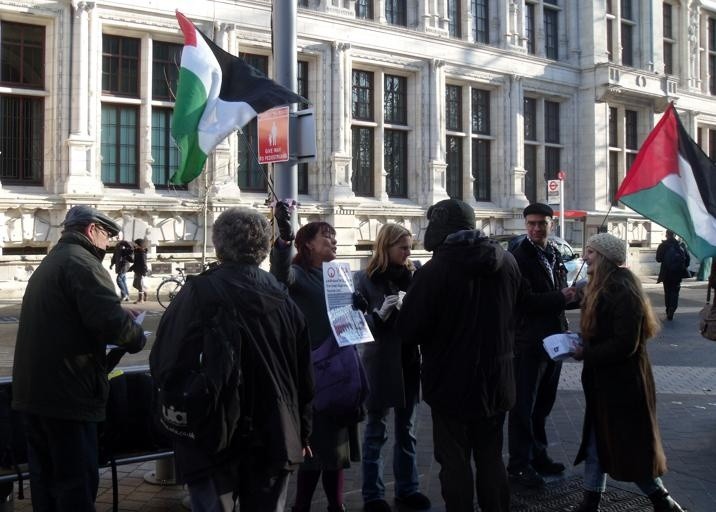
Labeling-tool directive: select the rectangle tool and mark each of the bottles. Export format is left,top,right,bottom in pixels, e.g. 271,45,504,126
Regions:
420,198,476,251
585,232,627,266
522,203,554,219
59,205,122,237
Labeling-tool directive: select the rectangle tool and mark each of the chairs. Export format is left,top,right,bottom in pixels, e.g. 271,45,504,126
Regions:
362,488,433,511
507,443,566,491
120,294,130,301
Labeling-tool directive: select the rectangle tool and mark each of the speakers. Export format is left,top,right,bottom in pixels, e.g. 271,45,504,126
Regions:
570,490,603,511
647,486,686,512
133,288,148,304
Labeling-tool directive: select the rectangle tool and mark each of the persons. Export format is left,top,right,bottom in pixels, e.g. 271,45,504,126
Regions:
562,232,686,512
9,203,146,511
505,203,579,483
655,229,690,320
148,205,315,511
353,224,431,512
268,198,372,512
390,199,521,511
109,231,133,301
132,239,148,304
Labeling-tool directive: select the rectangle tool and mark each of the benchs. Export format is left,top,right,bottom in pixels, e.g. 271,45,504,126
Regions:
613,101,716,262
168,9,309,189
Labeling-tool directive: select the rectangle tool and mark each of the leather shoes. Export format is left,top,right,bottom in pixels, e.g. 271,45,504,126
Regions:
351,289,369,314
375,294,399,323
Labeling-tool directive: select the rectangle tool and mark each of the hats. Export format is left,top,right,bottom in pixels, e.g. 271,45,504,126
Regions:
490,232,589,287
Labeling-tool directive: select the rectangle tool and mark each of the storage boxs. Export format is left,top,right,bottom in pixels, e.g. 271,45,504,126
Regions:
0,365,176,512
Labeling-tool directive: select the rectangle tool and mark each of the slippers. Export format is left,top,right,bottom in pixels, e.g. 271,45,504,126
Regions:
661,237,688,275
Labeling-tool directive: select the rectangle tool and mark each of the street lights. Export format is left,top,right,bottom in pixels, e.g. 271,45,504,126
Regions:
698,276,716,341
152,271,250,457
307,327,364,417
